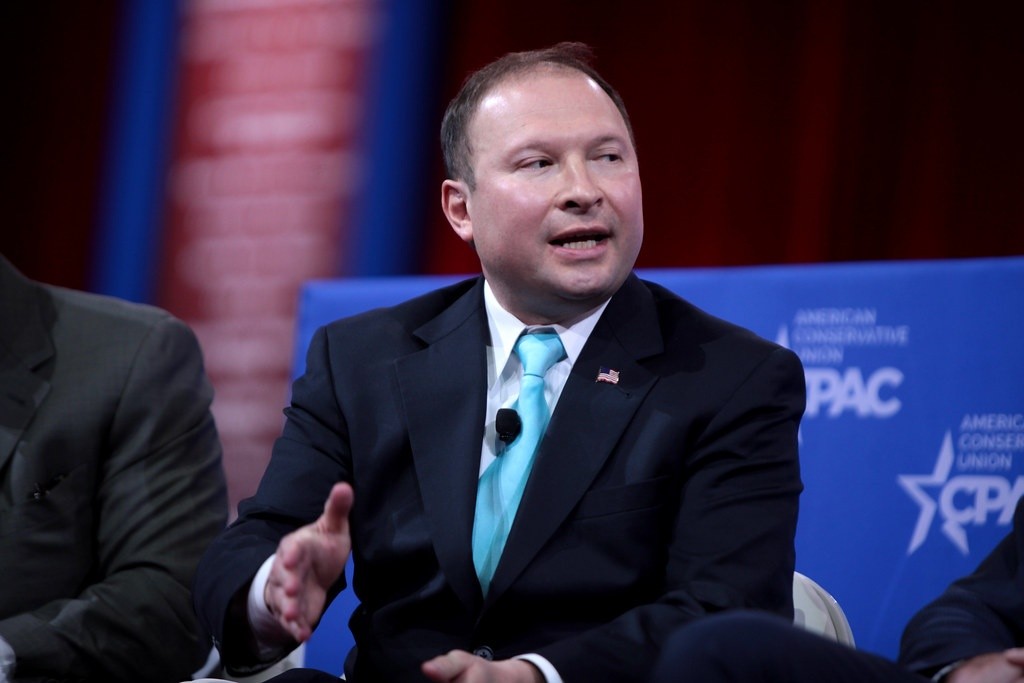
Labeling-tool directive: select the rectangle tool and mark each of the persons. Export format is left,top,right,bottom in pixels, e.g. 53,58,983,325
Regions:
653,491,1024,683
0,255,229,683
191,43,806,683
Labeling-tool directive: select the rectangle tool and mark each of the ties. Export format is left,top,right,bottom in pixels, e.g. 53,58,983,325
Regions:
469,329,568,598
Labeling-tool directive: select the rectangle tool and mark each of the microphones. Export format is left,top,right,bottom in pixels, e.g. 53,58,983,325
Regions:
495,409,521,441
